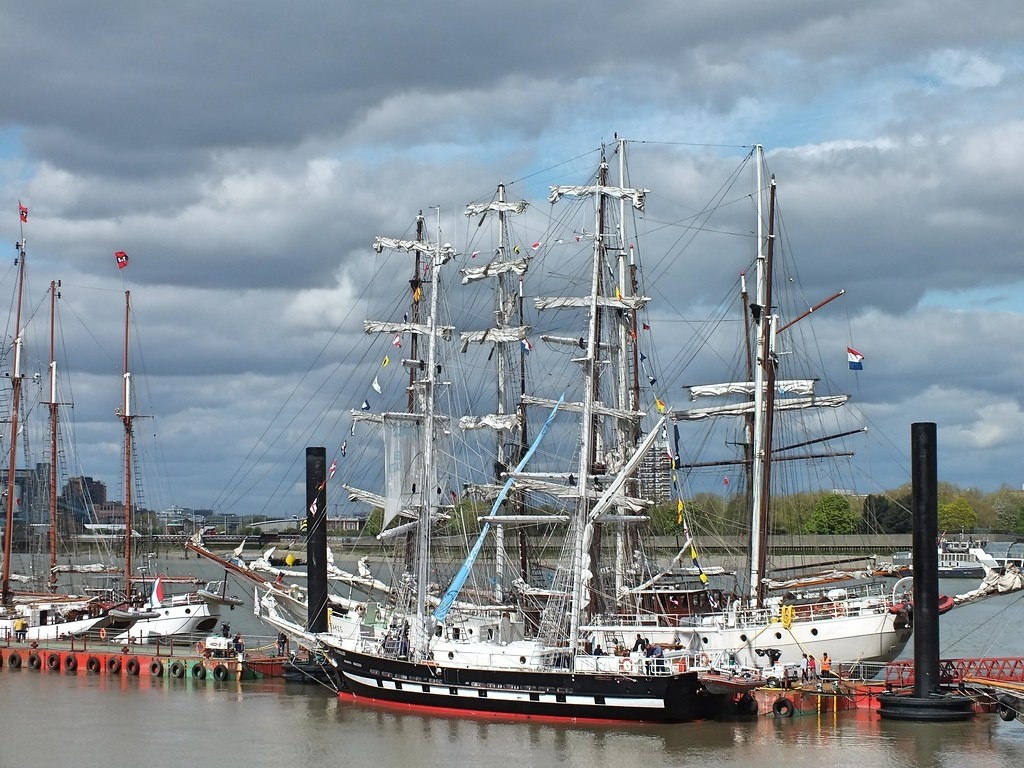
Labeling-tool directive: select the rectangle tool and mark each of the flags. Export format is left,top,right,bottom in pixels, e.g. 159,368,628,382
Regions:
848,347,865,370
18,200,28,222
115,251,129,270
151,575,164,609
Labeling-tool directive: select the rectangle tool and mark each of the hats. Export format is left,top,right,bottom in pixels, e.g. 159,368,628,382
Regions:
823,653,828,655
23,618,26,621
637,634,641,636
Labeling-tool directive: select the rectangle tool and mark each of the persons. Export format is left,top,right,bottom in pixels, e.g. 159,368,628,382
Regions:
276,630,287,656
808,655,817,681
643,644,666,676
594,644,606,655
13,617,28,643
634,633,645,652
821,653,831,682
133,592,145,611
22,618,30,643
801,653,808,683
233,633,244,658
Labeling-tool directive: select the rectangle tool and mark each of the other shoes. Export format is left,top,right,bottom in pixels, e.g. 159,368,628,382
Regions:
22,640,24,643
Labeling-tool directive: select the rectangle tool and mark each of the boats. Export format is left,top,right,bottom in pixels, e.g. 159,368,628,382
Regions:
222,555,301,566
892,538,1005,578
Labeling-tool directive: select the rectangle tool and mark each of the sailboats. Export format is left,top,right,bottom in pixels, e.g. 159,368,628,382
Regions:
0,252,225,641
183,135,1024,723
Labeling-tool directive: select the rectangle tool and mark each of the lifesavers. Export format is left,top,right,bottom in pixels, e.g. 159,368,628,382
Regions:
772,697,795,719
99,627,107,640
169,661,185,678
126,658,140,675
0,652,21,668
698,652,710,665
27,654,41,669
619,658,635,672
212,664,229,682
46,654,60,670
191,663,206,680
997,695,1016,722
196,641,206,654
149,660,164,677
64,655,78,672
85,657,101,672
107,657,121,674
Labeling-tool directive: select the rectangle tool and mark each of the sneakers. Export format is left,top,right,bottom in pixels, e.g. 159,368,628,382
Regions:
799,682,805,685
16,639,21,643
806,682,812,685
